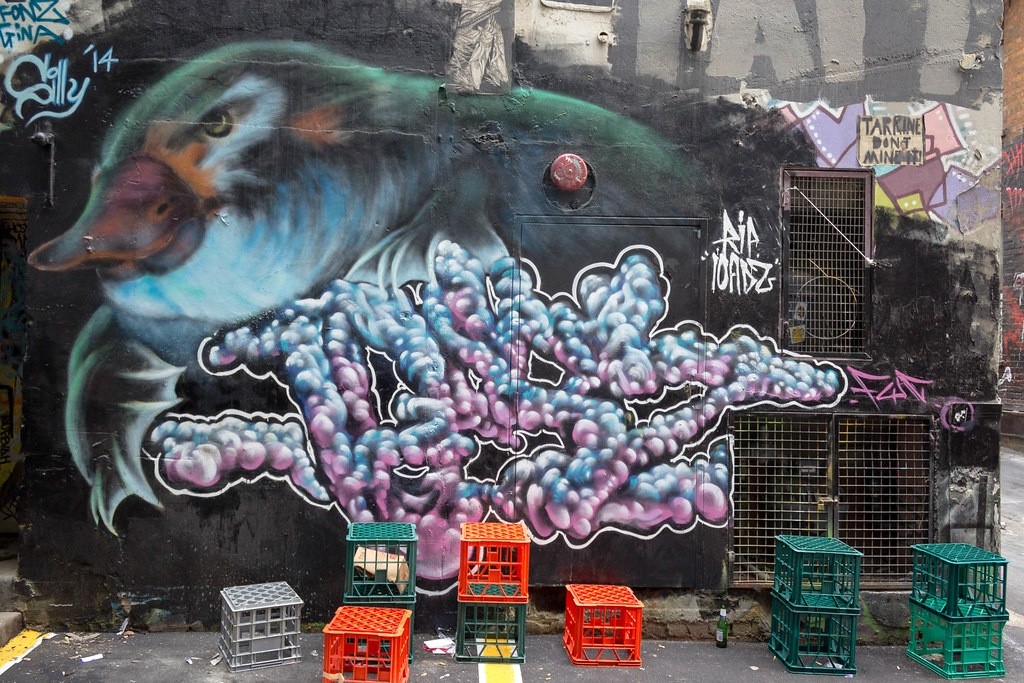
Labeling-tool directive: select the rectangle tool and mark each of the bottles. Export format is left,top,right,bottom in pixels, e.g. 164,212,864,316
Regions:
715,605,728,648
